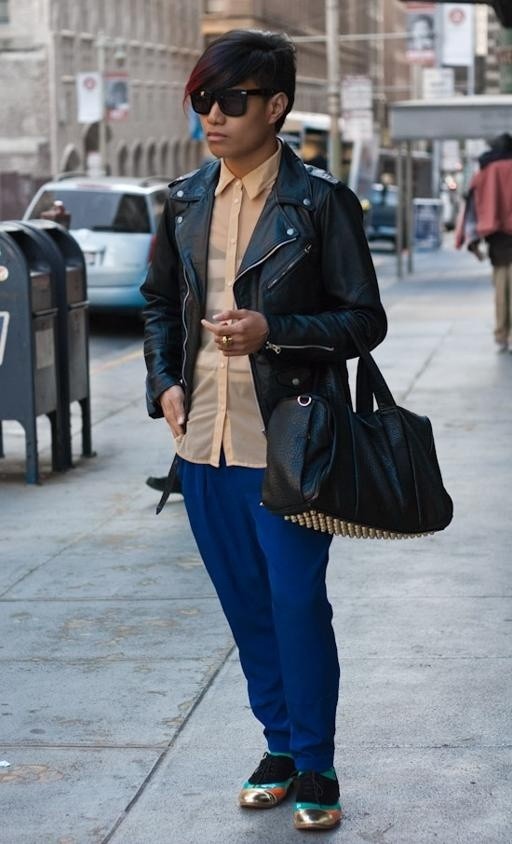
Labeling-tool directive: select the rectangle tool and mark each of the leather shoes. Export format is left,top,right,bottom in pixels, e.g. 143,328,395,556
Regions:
292,766,341,829
239,751,296,809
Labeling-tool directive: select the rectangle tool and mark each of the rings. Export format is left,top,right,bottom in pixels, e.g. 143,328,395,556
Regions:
222,335,233,348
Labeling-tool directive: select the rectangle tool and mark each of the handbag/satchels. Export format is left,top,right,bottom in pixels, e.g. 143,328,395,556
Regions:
259,311,454,540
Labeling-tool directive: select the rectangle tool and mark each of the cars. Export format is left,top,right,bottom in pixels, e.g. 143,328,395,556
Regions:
20,171,173,338
361,183,407,253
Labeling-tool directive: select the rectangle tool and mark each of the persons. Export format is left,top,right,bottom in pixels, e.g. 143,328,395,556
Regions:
453,132,512,355
406,13,432,51
139,28,389,831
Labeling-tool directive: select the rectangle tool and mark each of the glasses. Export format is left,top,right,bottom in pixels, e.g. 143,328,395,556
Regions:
189,85,273,116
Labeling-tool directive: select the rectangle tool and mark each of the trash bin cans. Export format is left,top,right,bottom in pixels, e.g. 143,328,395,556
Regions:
1,218,92,485
415,198,443,250
